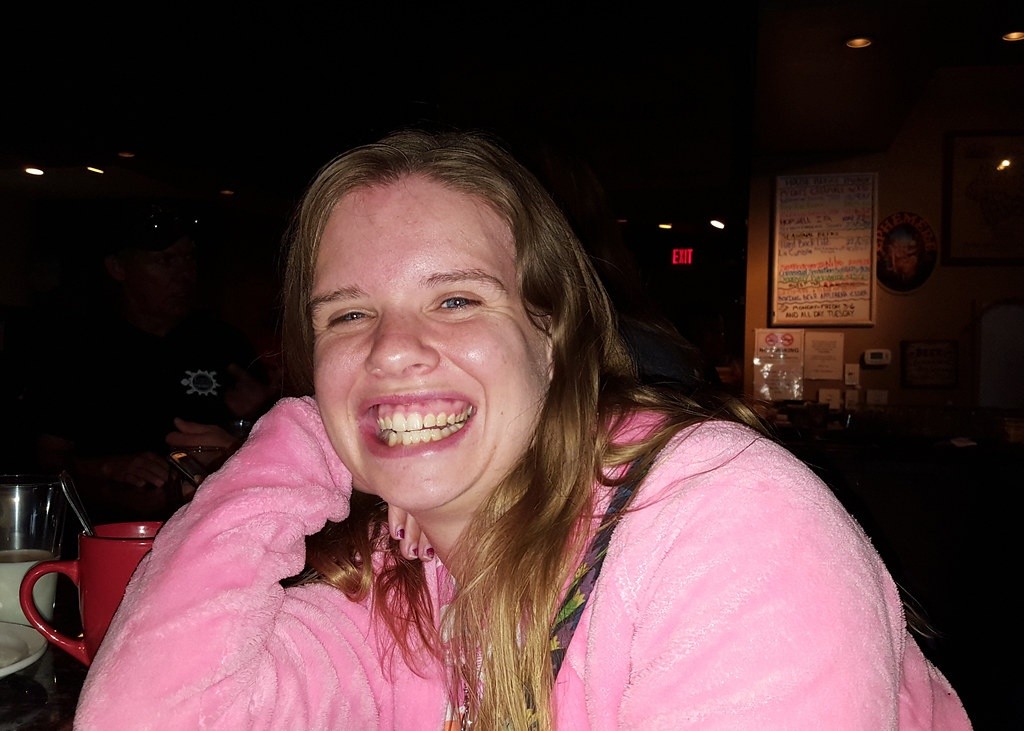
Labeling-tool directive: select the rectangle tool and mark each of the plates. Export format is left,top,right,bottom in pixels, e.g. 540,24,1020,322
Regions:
0,621,48,679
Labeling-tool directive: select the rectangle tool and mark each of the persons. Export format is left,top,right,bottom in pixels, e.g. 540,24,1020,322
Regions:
10,197,282,489
71,128,977,731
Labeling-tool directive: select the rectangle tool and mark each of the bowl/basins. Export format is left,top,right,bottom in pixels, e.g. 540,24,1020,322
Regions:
787,403,829,426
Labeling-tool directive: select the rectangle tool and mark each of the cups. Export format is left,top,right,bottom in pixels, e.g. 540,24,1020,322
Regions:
0,549,61,627
0,473,67,559
19,521,165,668
